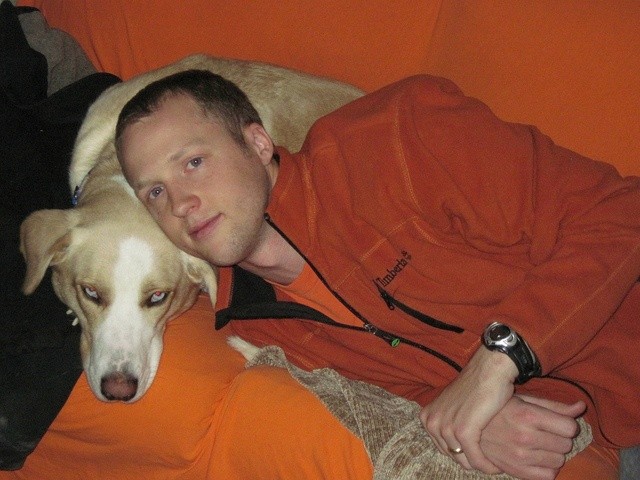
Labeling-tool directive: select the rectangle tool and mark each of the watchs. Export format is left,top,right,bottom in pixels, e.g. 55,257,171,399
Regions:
480,321,542,384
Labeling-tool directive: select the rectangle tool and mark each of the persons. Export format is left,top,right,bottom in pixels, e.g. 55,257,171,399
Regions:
115,70,640,480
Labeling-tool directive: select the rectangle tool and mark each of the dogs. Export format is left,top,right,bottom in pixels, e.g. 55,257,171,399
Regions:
19,53,369,404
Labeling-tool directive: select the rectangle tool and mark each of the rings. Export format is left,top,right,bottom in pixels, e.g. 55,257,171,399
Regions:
447,447,464,454
